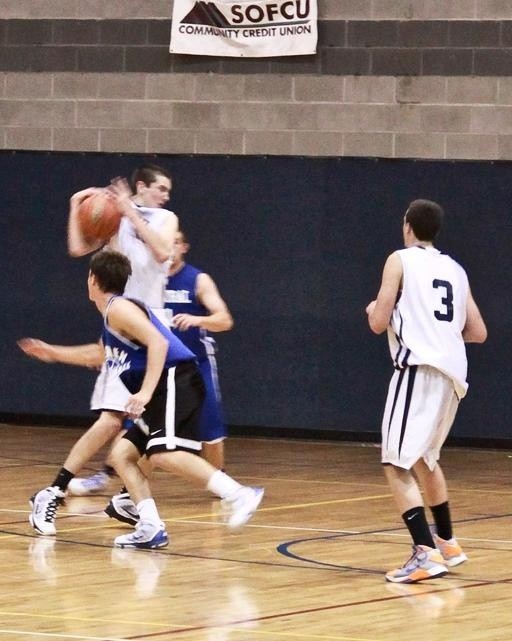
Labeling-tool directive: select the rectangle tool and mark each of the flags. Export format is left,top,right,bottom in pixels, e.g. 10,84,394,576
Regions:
168,0,319,59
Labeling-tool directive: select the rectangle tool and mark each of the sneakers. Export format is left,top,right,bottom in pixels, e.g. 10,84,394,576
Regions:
386,545,449,583
29,486,68,536
229,487,265,529
104,492,140,525
112,519,167,550
67,470,111,496
432,533,468,567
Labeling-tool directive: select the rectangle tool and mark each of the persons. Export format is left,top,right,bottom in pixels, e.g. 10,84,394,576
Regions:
25,535,71,640
16,244,267,553
108,544,163,602
27,163,180,535
67,227,236,498
384,578,465,641
364,200,488,585
200,502,265,641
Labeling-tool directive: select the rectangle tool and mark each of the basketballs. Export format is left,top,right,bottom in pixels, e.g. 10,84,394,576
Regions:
77,196,121,241
